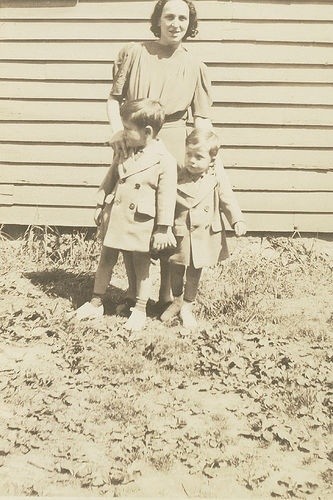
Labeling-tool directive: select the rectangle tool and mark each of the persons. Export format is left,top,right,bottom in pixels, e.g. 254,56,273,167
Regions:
107,0,214,315
74,98,178,331
159,128,247,331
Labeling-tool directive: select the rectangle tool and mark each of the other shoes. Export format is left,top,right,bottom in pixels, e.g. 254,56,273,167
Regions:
180,300,196,330
116,296,135,317
74,302,104,319
124,308,147,330
160,292,184,321
